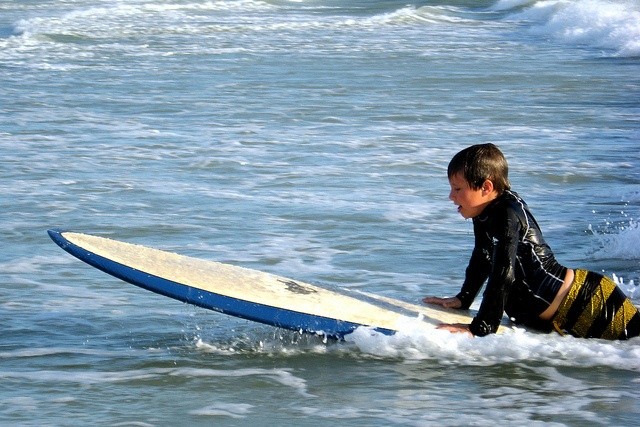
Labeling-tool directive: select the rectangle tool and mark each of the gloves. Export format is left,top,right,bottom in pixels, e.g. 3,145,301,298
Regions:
44,222,558,351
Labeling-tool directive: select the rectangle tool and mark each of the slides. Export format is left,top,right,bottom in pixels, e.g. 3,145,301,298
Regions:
46,226,543,344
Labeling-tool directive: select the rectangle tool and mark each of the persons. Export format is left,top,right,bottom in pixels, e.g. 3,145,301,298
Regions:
423,143,640,342
